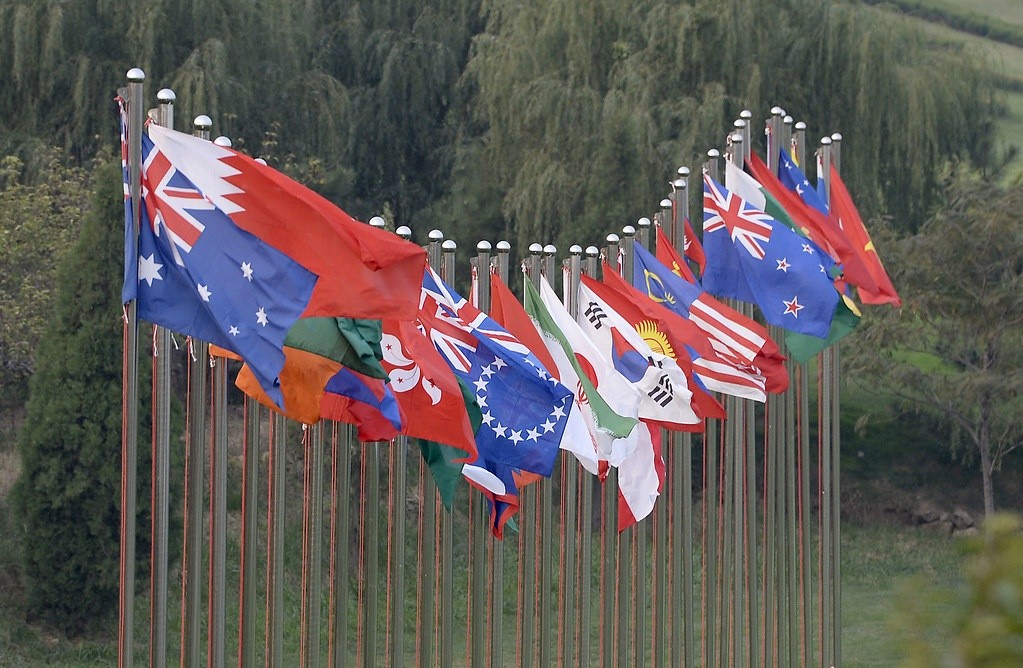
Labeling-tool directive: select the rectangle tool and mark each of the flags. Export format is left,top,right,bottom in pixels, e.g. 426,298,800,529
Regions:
116,103,902,540
